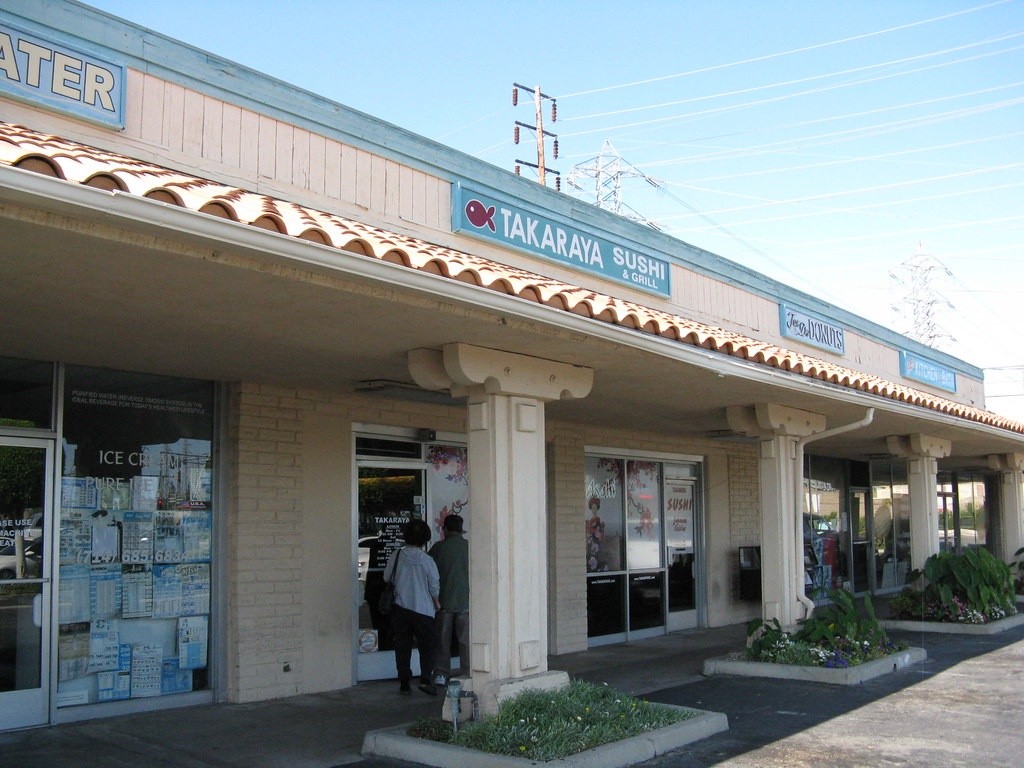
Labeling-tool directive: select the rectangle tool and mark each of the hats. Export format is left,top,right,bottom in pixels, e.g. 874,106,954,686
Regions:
444,514,463,529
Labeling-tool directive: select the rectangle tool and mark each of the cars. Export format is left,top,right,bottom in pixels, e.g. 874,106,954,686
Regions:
800,514,866,561
0,511,47,581
356,533,404,580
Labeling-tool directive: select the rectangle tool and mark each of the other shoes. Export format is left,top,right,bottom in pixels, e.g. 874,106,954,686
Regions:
418,683,437,695
434,674,447,686
399,684,410,694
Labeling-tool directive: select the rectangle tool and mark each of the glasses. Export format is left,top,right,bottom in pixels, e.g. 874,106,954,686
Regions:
442,526,447,531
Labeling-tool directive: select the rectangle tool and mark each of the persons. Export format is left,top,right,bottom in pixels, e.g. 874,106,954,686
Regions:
430,515,470,687
383,519,441,696
585,500,609,573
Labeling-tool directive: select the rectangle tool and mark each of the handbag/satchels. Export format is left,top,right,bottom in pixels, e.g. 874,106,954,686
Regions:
370,582,395,626
